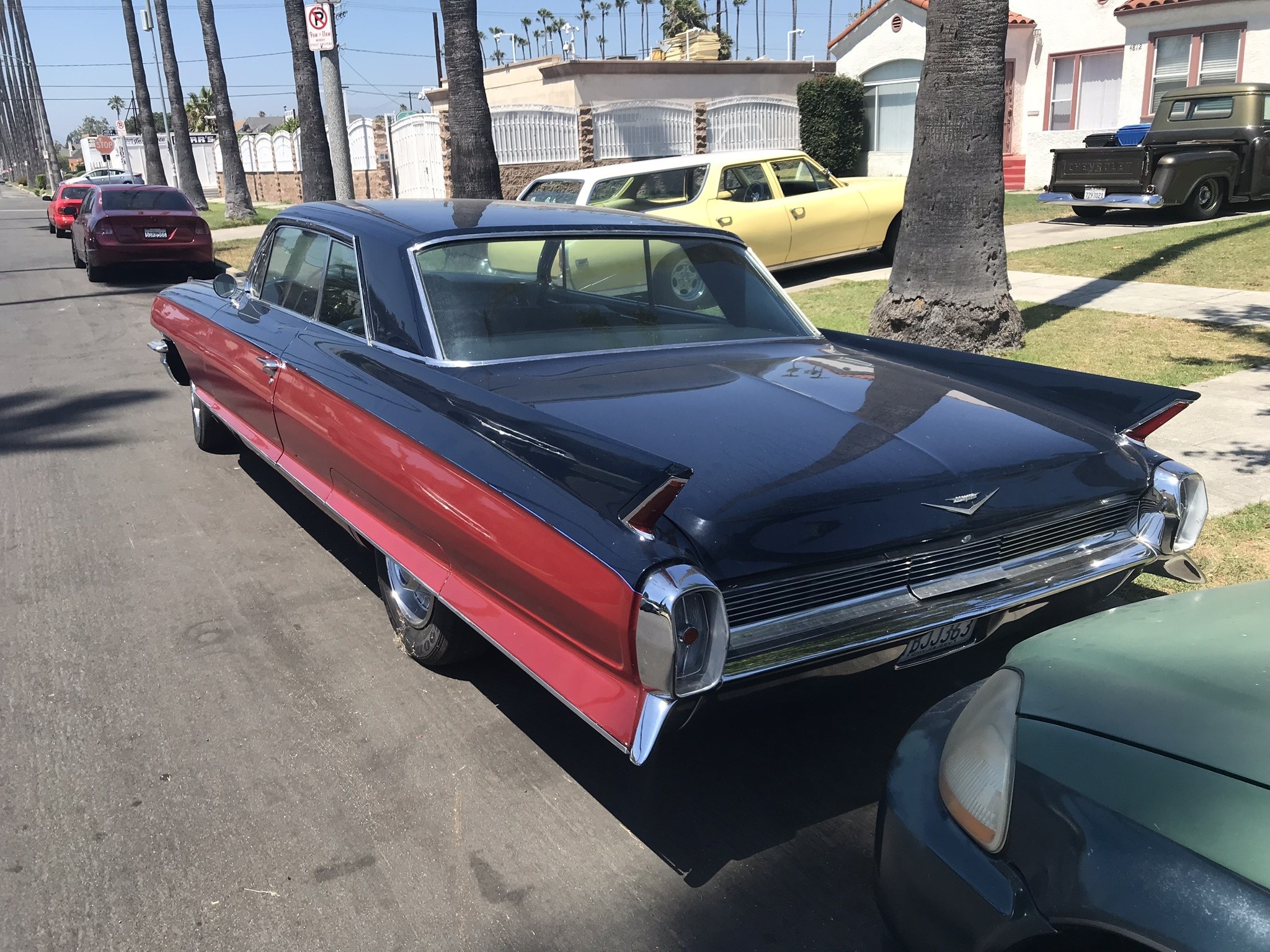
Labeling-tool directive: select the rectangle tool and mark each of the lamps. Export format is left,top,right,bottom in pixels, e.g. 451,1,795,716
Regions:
1033,29,1044,47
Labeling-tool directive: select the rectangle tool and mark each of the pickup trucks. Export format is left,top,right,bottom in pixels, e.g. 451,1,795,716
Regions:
1037,83,1270,222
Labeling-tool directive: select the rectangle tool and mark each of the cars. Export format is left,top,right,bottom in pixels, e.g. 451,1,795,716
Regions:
873,581,1270,952
0,178,5,183
148,197,1209,768
42,183,98,238
56,167,145,187
61,182,213,283
416,149,907,302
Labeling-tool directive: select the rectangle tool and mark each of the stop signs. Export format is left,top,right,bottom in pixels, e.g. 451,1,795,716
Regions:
94,135,115,155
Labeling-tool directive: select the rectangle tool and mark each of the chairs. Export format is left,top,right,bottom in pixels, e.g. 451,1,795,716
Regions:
426,271,622,340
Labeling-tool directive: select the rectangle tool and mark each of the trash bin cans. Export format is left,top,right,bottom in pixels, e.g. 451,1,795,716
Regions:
1115,124,1152,146
1083,133,1115,147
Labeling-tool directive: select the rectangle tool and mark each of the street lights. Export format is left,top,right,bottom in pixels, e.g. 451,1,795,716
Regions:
686,28,699,61
787,29,805,61
494,32,517,63
541,40,554,57
561,23,580,60
